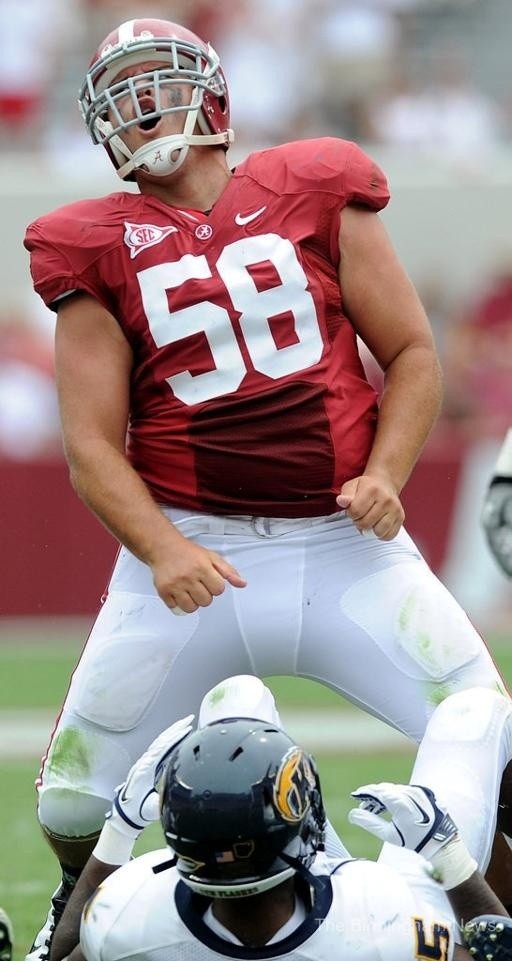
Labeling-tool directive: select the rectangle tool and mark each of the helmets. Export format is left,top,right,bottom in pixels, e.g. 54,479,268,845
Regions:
153,714,329,903
79,16,234,180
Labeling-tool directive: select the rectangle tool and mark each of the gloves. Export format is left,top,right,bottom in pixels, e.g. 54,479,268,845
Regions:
89,707,201,872
345,777,483,897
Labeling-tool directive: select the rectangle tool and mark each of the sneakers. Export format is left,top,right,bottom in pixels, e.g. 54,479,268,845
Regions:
17,879,77,959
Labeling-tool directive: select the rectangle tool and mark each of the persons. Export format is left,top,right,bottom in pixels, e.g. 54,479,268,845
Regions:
437,263,512,611
46,674,512,961
462,914,512,961
0,906,13,961
481,424,512,577
27,18,512,961
